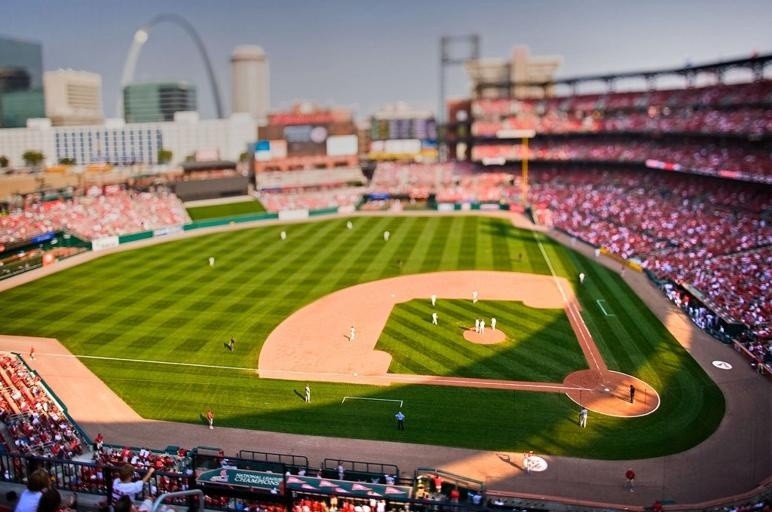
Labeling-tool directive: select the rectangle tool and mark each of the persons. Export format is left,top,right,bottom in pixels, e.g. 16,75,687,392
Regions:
621,500,664,512
349,325,355,341
228,334,235,353
577,406,587,428
471,89,769,384
701,489,772,512
432,311,438,326
0,350,506,512
629,384,635,403
523,450,533,475
622,467,635,493
0,160,538,276
431,292,436,306
